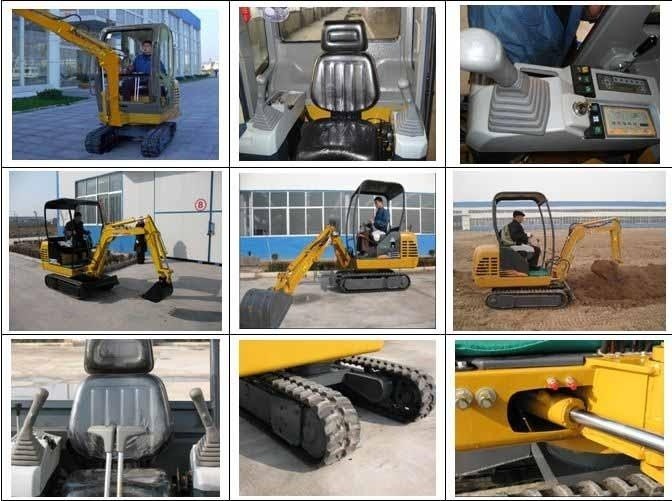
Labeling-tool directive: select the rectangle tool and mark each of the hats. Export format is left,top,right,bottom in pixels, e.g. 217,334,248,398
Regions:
513,209,526,217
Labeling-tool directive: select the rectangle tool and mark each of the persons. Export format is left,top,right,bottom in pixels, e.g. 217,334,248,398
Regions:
354,195,390,258
466,6,605,66
500,208,540,272
118,39,167,103
65,212,93,263
134,215,147,265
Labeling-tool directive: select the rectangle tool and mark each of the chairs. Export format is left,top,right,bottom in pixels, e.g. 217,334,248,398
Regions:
296,21,378,163
63,339,173,498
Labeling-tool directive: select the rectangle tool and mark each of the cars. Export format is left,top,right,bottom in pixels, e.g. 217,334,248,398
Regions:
345,8,364,21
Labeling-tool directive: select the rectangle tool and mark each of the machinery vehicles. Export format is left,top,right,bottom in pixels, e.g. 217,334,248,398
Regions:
470,191,623,310
38,197,177,303
13,9,183,158
241,178,420,331
238,338,438,469
456,342,664,497
